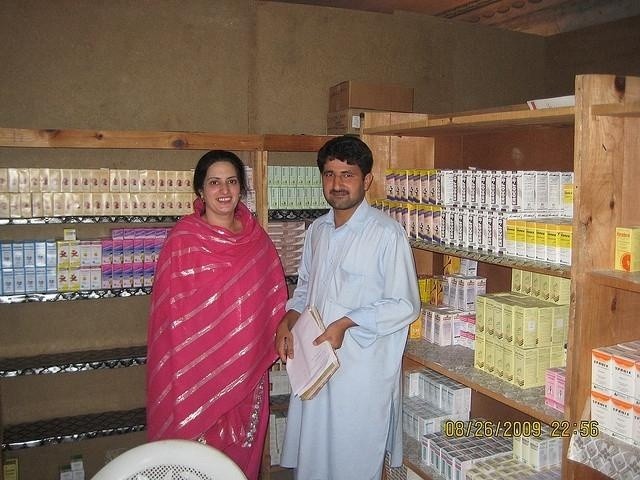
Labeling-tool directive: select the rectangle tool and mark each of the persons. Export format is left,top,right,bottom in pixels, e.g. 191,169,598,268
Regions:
272,136,421,479
144,150,291,479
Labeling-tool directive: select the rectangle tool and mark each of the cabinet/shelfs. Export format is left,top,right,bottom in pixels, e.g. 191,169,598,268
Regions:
359,73,579,480
0,127,262,479
262,134,343,480
570,75,639,480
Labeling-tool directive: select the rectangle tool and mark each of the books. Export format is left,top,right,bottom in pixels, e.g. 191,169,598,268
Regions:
285,304,340,401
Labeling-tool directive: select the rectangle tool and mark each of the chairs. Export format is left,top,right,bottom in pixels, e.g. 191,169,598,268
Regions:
90,439,248,479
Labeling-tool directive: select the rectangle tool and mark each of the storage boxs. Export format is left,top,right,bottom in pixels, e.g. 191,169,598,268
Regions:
326,109,365,136
330,80,414,111
